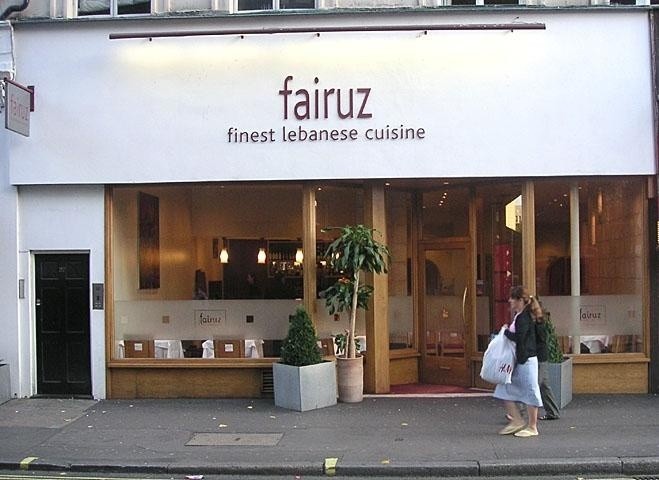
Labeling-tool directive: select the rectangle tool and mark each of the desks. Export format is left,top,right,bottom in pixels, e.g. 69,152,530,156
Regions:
203,339,265,358
119,339,183,358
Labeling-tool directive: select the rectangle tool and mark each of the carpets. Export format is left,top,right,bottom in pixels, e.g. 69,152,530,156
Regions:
391,383,482,393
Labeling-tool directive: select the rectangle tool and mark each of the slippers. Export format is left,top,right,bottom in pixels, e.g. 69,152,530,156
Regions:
513,426,540,437
497,419,528,435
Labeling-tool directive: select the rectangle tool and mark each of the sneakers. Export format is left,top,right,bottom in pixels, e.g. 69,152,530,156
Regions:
504,413,514,422
538,413,561,420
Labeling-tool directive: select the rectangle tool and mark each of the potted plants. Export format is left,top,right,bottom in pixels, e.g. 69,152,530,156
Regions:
321,225,390,404
543,315,573,409
271,307,337,412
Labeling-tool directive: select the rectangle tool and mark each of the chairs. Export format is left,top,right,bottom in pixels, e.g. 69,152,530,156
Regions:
555,334,633,354
123,339,155,359
212,339,245,358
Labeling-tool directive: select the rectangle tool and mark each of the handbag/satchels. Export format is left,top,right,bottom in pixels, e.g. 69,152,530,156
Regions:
477,325,518,386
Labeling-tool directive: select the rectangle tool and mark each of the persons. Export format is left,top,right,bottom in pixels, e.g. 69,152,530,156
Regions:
505,297,561,422
243,271,265,299
491,284,545,437
